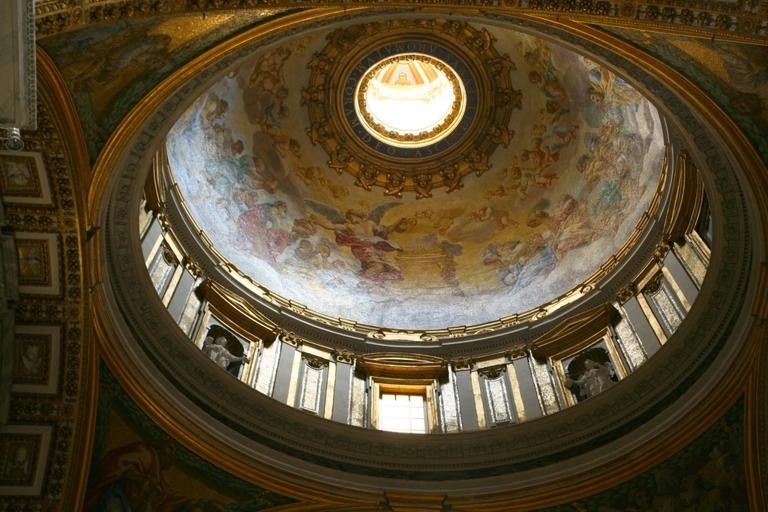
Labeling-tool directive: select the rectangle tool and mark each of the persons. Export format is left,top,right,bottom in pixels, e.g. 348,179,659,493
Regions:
206,337,250,372
730,90,768,141
563,358,614,396
81,434,187,512
626,485,658,512
87,35,173,119
